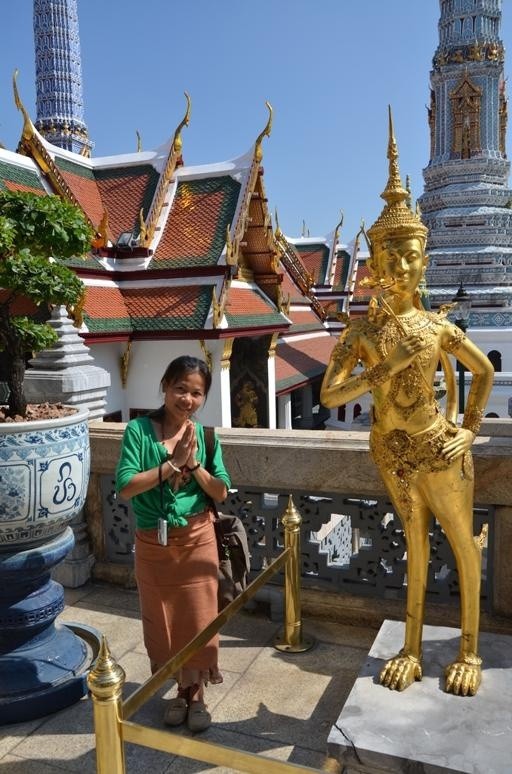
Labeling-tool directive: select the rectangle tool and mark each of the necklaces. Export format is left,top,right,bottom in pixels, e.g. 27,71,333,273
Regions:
159,405,189,446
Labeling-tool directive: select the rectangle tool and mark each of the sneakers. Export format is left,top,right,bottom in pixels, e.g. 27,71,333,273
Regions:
163,692,212,731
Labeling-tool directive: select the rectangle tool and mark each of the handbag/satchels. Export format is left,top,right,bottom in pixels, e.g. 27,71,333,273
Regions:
214,512,251,596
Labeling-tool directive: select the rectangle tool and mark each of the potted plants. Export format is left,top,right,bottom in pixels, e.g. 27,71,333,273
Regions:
0,182,94,548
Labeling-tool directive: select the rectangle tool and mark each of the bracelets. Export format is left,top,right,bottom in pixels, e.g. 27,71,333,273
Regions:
188,463,200,472
366,360,393,389
167,459,181,473
459,405,485,435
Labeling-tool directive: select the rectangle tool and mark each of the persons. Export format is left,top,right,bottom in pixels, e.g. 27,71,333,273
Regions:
321,236,497,697
112,353,232,736
234,377,259,427
